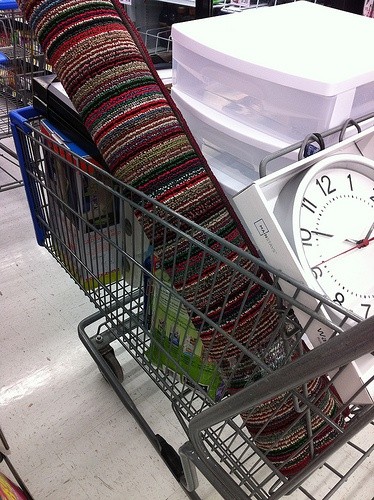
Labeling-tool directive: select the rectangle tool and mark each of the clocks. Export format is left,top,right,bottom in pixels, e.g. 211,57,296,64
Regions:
273,152,373,328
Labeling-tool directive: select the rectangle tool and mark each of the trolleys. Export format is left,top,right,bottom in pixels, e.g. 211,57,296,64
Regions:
6,104,374,500
0,0,48,192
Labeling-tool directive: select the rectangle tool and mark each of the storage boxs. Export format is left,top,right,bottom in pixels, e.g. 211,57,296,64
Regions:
171,1,374,199
40,118,121,291
